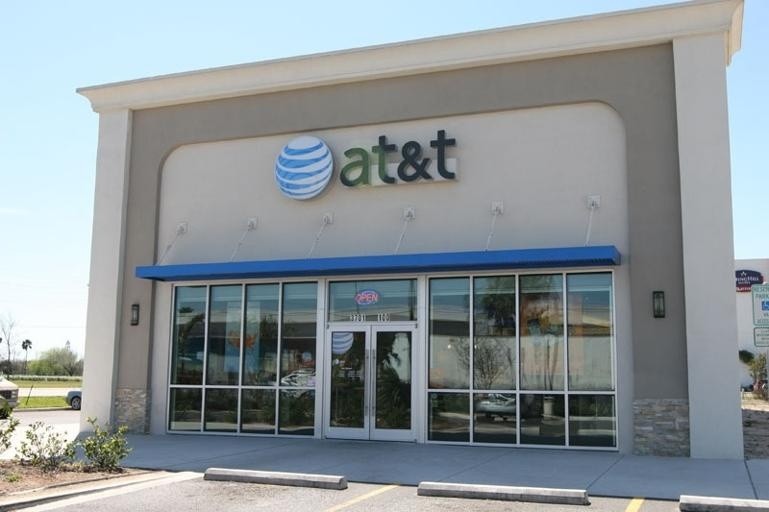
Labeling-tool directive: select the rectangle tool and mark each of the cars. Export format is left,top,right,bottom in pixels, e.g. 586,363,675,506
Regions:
473,392,531,424
63,388,81,411
740,379,768,393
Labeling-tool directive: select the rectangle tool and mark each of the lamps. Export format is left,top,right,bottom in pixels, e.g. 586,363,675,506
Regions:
652,290,666,319
130,302,140,327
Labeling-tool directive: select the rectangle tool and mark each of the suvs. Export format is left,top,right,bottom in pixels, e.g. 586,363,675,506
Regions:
1,371,20,419
176,355,210,402
